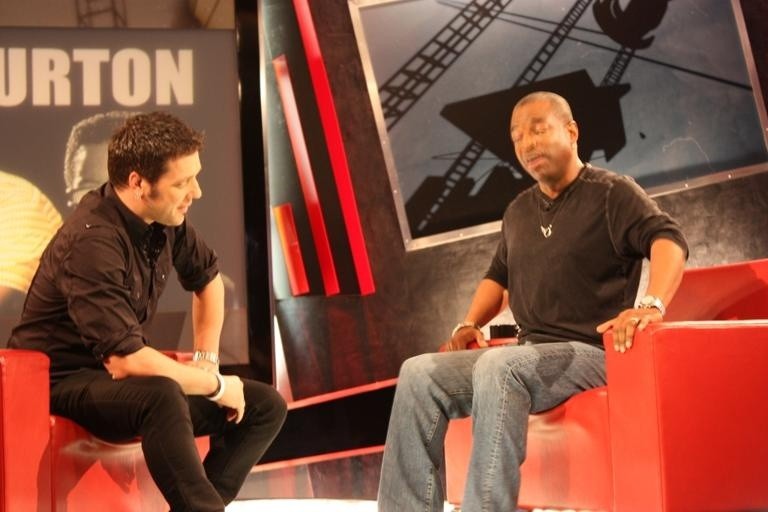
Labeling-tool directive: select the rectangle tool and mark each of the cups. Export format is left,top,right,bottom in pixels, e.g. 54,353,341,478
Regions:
490,324,519,340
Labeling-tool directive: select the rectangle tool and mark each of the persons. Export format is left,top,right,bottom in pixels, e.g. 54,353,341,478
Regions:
7,111,287,511
63,110,137,212
377,92,689,511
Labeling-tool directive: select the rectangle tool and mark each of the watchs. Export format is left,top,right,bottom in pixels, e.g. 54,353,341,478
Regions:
637,295,665,316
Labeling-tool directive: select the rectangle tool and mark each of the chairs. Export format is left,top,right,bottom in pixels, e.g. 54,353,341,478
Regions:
0,349,211,512
439,259,767,511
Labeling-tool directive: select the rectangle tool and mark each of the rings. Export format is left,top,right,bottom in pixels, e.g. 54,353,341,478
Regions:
632,318,638,323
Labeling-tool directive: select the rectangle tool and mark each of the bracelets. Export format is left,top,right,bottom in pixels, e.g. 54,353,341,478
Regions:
193,351,218,365
206,374,225,403
452,321,480,337
